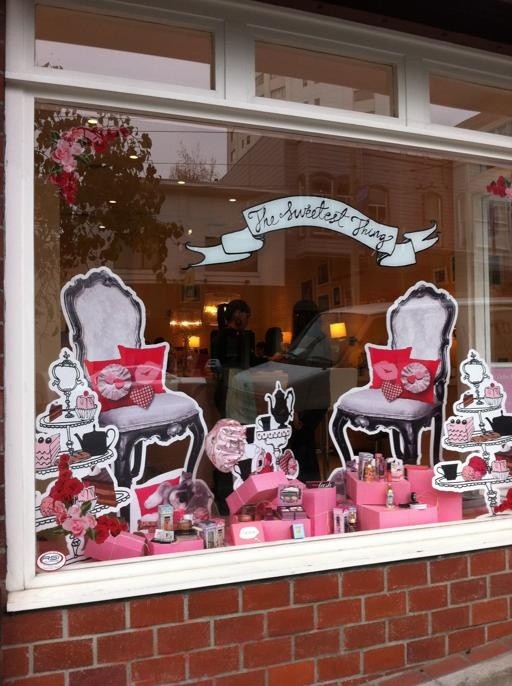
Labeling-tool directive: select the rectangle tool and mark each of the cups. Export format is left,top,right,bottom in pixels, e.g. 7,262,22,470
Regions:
258,416,271,432
233,458,252,481
436,463,458,480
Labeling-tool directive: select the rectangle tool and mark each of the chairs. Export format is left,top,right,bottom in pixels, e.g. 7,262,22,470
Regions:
327,280,458,468
59,265,208,531
326,366,358,454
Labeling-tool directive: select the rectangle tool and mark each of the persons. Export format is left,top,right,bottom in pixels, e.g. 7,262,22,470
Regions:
210,300,329,516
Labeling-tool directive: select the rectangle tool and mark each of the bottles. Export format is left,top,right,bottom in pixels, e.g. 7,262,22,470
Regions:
387,489,394,509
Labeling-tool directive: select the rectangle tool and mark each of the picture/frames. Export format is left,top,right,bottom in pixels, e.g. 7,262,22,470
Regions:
299,259,342,312
178,284,201,304
432,267,448,286
450,255,455,284
487,253,503,287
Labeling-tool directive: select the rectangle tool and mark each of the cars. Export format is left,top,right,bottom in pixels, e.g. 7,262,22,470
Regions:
226,298,512,425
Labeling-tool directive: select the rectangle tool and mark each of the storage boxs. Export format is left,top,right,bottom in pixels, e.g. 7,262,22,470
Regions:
82,465,463,562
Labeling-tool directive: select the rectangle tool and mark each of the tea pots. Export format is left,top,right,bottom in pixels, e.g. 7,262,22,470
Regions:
485,410,512,436
265,381,295,429
75,424,116,456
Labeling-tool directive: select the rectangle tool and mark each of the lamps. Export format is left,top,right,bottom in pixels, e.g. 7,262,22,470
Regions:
281,331,292,344
188,336,200,348
330,322,348,339
